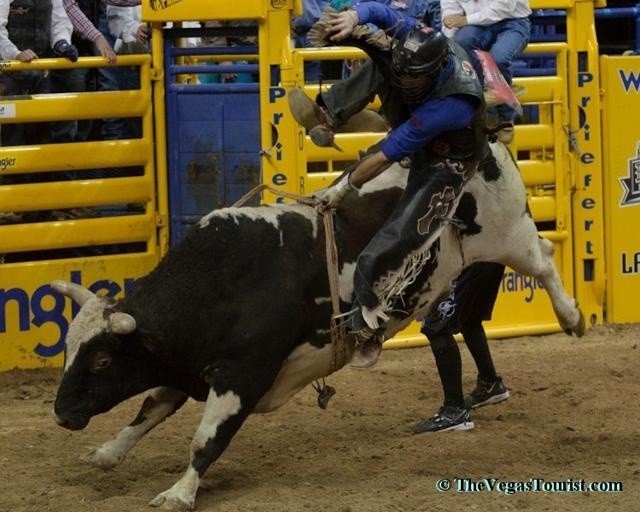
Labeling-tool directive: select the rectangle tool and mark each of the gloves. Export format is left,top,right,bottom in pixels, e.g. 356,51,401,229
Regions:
52,40,79,62
316,169,361,212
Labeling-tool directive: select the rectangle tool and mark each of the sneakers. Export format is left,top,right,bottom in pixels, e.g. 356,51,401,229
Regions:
410,406,475,437
464,375,510,409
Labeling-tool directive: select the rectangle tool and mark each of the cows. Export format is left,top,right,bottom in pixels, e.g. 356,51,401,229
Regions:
52,85,587,512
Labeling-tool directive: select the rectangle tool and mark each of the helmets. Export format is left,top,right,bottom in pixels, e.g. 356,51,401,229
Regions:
391,24,453,104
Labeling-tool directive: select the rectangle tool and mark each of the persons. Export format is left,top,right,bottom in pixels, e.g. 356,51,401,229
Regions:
287,3,489,367
1,1,640,258
404,253,516,436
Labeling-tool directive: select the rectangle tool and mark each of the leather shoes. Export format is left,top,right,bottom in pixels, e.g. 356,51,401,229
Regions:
286,89,334,146
496,118,514,143
349,337,382,368
